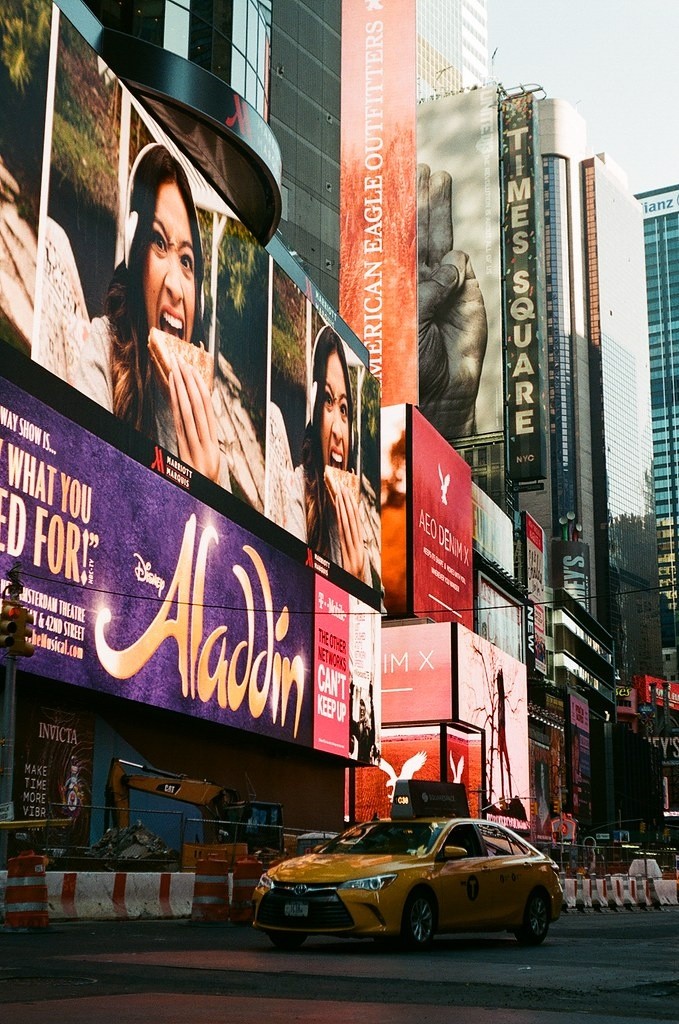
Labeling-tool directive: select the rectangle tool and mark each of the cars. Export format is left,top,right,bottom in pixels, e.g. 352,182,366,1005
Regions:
250,778,563,949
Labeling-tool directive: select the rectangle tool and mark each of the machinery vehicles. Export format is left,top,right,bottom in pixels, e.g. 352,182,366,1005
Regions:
105,758,287,873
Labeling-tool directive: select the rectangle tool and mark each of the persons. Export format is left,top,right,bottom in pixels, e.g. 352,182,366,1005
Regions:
1,139,389,598
350,700,375,760
419,163,487,440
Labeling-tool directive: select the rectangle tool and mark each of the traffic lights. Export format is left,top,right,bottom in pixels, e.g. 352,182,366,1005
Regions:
531,802,538,816
554,800,560,814
10,609,34,657
0,601,16,648
640,823,646,833
499,797,506,810
664,828,671,838
